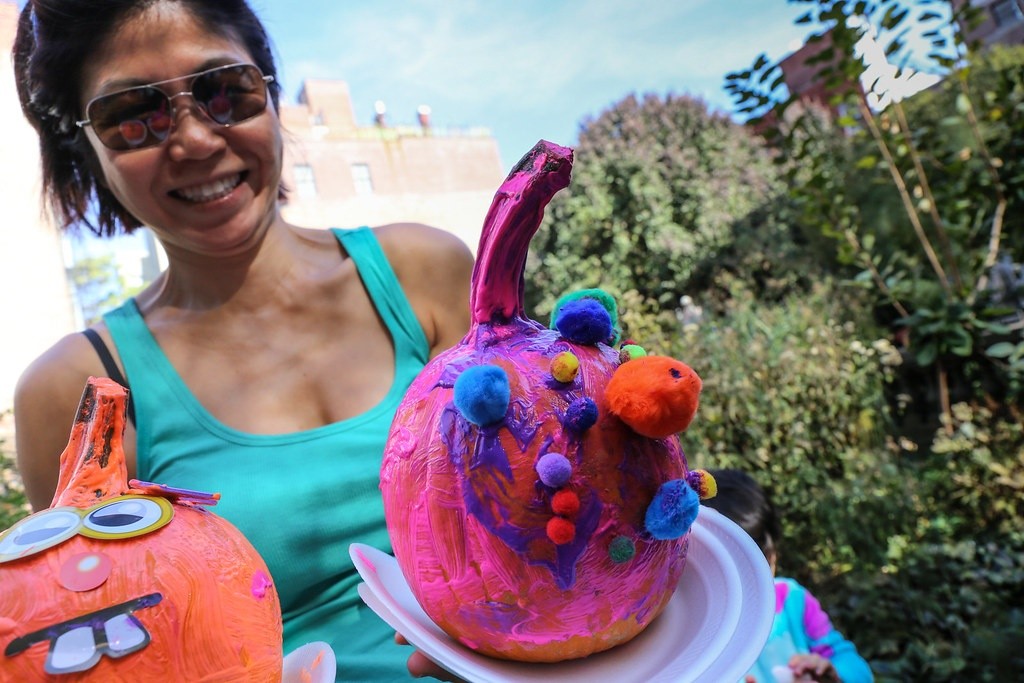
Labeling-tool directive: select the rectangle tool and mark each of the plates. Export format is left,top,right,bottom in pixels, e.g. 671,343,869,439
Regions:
347,503,776,683
282,641,337,683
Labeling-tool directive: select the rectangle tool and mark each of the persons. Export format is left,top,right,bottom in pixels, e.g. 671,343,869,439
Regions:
691,470,875,683
14,0,479,683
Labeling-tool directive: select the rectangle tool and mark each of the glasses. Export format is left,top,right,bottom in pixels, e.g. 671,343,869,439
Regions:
81,63,274,151
